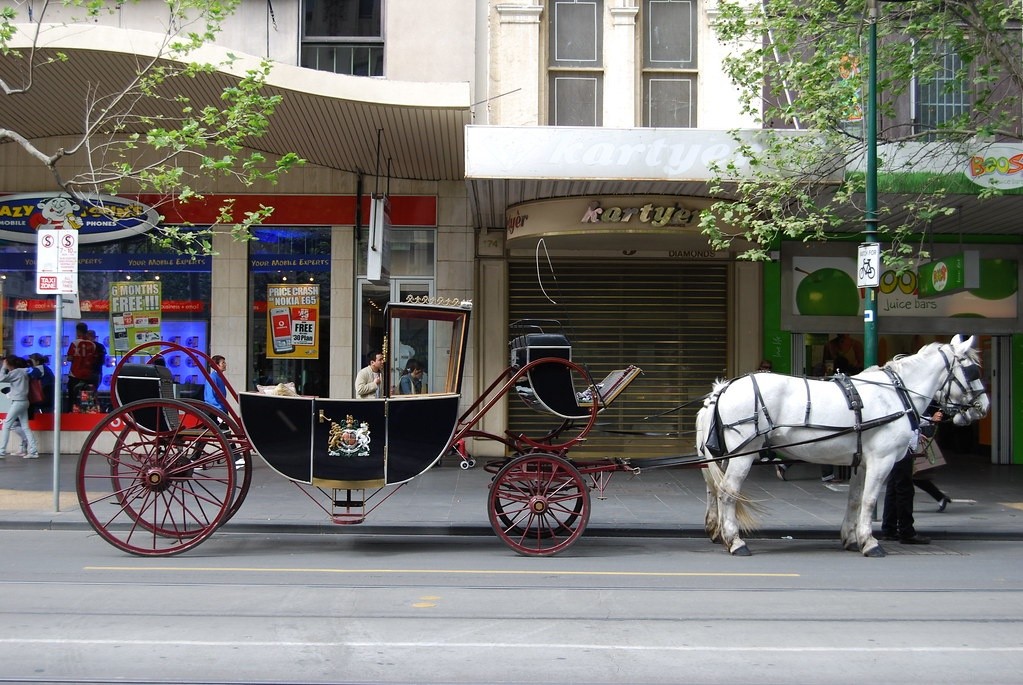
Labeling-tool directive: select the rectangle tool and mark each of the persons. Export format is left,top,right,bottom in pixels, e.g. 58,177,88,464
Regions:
454,435,468,458
881,412,951,543
759,360,837,481
400,359,423,394
0,353,55,458
355,351,393,398
67,322,107,411
190,355,245,470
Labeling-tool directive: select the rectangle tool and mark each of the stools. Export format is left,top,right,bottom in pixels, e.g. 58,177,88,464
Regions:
507,319,571,351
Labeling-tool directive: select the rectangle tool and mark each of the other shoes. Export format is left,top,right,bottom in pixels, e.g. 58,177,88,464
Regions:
0,455,6,458
190,460,203,470
882,530,901,541
235,458,245,470
10,451,27,456
901,534,931,544
828,477,843,482
775,464,786,480
23,453,39,459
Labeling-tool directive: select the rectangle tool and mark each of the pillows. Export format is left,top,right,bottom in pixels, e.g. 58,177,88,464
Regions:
256,381,297,396
272,384,303,398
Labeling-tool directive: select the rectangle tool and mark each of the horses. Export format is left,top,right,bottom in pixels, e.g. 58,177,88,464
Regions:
694,334,990,559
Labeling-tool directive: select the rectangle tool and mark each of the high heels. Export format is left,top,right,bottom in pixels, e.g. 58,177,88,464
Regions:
936,496,952,512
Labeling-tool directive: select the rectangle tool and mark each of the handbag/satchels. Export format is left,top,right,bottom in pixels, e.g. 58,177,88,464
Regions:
912,436,946,475
28,377,44,403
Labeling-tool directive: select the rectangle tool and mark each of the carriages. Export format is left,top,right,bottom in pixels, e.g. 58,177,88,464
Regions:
72,290,993,561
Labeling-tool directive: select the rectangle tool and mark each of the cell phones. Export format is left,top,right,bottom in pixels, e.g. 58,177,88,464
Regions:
270,307,295,354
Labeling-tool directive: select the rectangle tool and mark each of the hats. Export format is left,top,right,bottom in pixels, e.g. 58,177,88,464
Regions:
86,330,99,338
